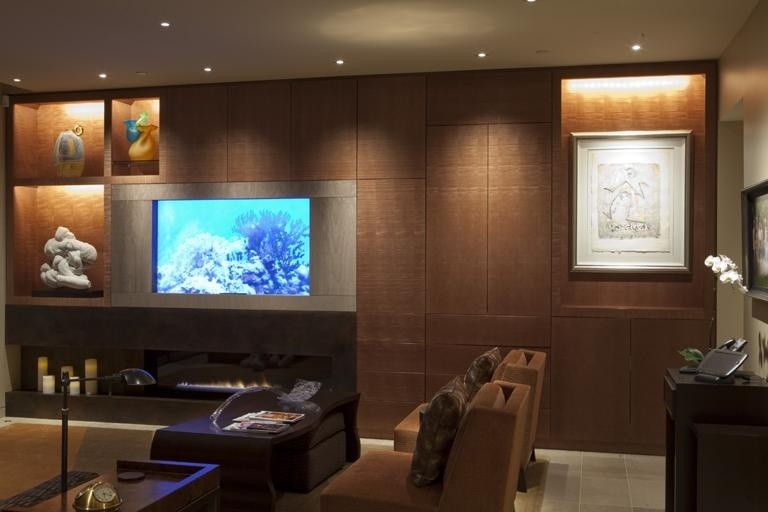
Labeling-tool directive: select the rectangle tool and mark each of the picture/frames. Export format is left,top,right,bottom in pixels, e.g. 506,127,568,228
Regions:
740,178,768,301
569,128,692,276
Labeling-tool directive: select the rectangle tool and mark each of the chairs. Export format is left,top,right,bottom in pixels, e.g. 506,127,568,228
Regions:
320,346,546,512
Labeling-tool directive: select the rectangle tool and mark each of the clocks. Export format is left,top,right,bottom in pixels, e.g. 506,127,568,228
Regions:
72,481,122,511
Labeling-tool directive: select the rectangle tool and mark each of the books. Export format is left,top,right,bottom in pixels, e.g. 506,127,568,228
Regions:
222,409,306,436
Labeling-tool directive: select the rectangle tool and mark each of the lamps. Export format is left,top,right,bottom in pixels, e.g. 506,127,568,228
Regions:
37,356,99,396
61,366,157,512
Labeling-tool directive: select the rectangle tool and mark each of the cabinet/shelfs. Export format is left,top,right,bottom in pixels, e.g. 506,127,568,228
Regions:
5,86,166,308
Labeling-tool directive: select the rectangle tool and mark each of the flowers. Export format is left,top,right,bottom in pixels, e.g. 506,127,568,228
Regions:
676,254,748,364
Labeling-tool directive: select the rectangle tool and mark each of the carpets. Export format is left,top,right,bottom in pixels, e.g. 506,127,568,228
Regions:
0,423,544,512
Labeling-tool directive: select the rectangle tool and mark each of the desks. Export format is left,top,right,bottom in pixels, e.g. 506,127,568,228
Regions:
663,367,767,512
0,458,223,511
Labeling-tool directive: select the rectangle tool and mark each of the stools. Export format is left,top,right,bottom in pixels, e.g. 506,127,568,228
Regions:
271,411,347,493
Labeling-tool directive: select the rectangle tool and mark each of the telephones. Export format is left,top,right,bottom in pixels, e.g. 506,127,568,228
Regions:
717,338,748,351
